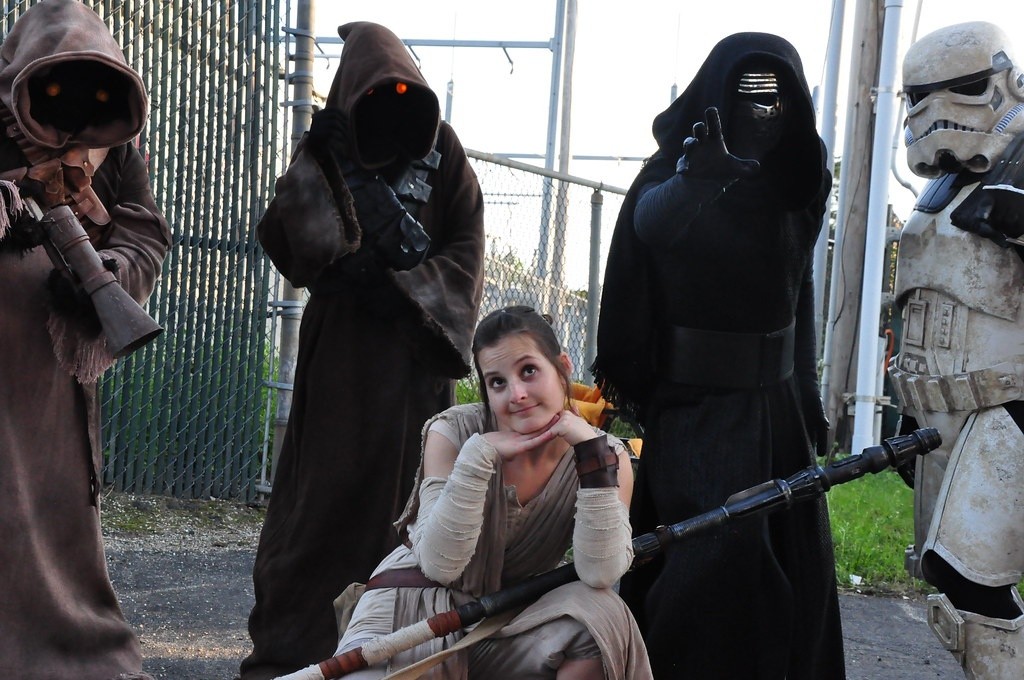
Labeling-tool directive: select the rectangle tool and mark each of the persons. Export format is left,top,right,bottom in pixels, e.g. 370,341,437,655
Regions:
326,305,655,679
238,14,487,680
0,0,175,680
586,31,845,679
878,21,1021,679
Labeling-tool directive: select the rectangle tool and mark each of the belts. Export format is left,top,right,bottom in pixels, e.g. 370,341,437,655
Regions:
362,566,458,597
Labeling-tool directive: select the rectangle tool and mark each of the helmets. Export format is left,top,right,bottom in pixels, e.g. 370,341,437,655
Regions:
894,22,1021,173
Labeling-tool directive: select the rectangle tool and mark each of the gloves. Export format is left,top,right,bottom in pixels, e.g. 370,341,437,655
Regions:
678,104,761,198
799,388,833,460
893,415,922,492
